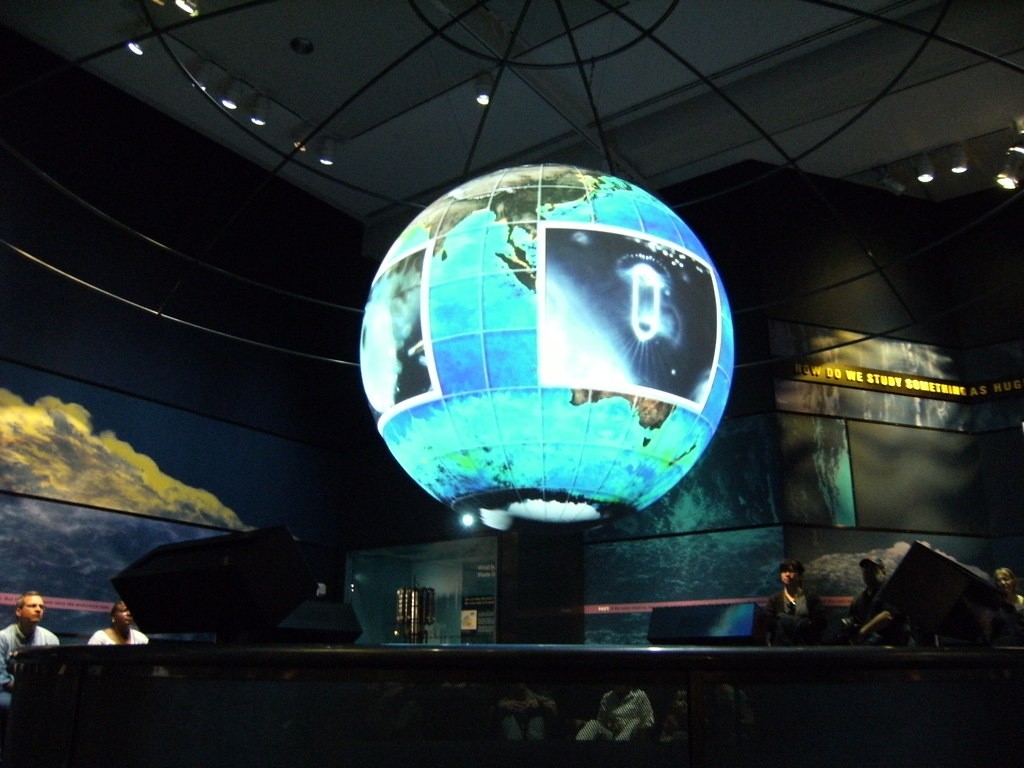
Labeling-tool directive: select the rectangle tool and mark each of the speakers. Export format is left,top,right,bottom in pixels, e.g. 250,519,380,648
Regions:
111,525,363,648
495,531,588,644
879,540,1002,645
648,601,771,647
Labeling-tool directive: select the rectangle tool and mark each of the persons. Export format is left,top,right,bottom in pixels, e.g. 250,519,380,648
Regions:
763,560,827,648
493,681,557,744
0,589,60,712
660,699,711,744
837,554,1024,650
87,600,150,646
574,678,655,742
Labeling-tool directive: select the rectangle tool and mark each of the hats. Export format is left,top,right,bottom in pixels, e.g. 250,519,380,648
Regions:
859,554,885,570
